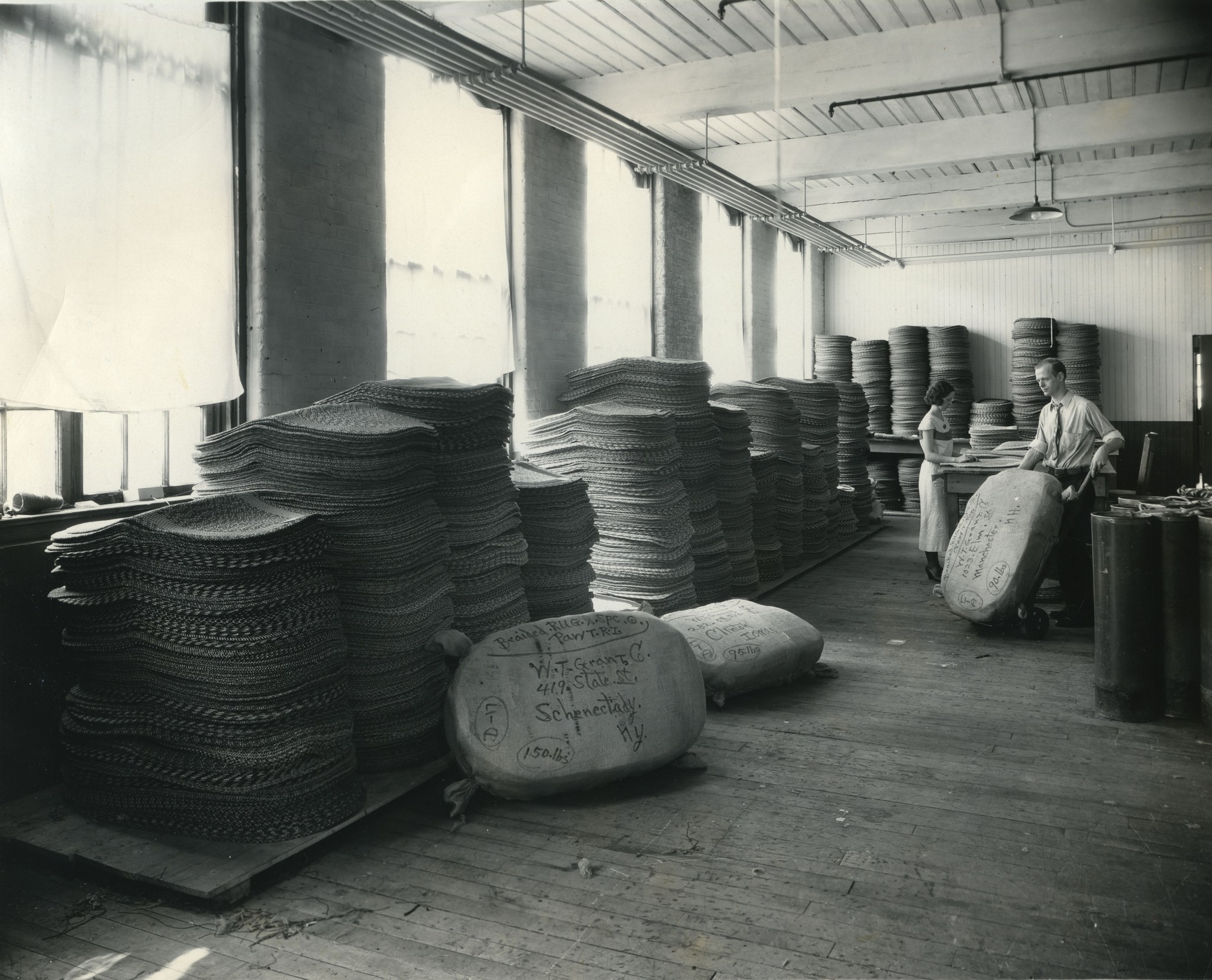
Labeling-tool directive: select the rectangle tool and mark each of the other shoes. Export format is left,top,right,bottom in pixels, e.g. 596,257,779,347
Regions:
1054,613,1094,628
1048,608,1067,620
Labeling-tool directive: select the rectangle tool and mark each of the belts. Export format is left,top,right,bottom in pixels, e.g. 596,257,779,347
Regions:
1045,465,1090,477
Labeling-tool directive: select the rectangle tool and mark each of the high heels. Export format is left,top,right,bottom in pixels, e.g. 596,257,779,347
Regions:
926,565,942,582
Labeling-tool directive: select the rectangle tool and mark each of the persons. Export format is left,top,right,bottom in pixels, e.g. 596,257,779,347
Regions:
917,380,981,585
1016,357,1124,628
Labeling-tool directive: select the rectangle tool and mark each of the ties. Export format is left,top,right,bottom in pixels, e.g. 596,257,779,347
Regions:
1051,403,1062,462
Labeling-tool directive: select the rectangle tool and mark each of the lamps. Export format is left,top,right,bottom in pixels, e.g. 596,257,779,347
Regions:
1008,157,1064,222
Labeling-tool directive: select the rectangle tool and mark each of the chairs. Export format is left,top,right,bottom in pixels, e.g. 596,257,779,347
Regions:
1105,431,1159,504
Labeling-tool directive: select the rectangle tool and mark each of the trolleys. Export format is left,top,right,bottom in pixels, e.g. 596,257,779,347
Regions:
934,464,1108,641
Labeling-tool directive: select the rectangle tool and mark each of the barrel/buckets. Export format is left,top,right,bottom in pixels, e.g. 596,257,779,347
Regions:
1090,494,1212,733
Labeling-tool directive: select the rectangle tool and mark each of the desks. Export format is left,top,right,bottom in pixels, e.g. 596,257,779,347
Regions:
937,448,1117,552
868,439,1126,502
